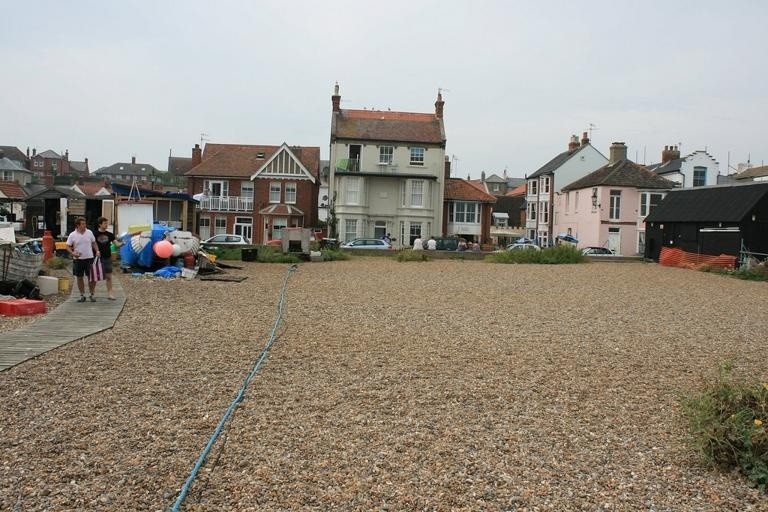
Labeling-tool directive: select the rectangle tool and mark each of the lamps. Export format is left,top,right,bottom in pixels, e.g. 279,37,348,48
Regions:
591,191,601,208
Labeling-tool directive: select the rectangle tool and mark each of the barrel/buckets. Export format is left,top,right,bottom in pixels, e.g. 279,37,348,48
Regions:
59,278,70,294
175,257,184,268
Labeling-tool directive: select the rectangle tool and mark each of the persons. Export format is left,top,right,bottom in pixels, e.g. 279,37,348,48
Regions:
412,234,424,250
93,215,128,300
64,217,102,303
472,240,481,251
457,238,468,251
426,235,437,250
383,232,391,244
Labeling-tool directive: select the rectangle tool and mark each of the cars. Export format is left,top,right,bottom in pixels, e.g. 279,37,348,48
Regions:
338,238,396,251
405,237,460,250
200,234,250,245
492,243,543,254
579,247,624,257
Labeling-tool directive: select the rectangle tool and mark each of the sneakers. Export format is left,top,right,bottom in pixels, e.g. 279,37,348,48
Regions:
90,294,96,302
108,294,116,300
77,296,86,302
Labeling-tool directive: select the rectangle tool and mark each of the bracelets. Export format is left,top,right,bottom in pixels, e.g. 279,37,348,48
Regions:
96,251,101,254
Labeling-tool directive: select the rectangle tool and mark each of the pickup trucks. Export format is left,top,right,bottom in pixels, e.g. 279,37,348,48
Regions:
0,214,26,233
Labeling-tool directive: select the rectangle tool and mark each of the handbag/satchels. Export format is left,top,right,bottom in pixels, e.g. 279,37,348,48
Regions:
89,263,106,282
